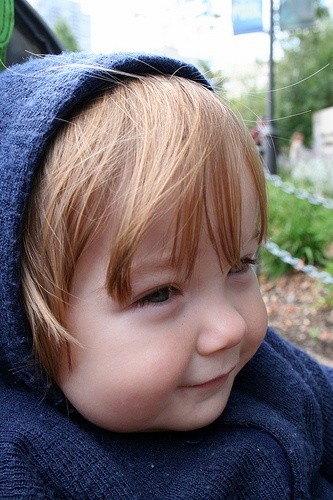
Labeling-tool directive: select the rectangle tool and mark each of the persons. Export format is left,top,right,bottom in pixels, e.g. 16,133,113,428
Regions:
286,127,315,177
254,113,276,174
0,44,333,499
